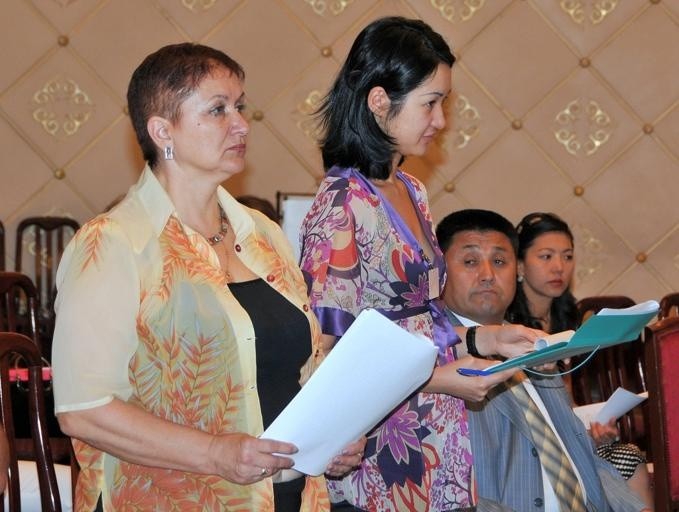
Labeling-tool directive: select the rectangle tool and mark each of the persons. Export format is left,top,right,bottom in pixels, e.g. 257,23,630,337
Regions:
434,207,652,511
298,16,572,512
52,43,368,512
502,212,656,512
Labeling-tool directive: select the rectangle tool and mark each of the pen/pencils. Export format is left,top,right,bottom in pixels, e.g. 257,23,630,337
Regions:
454,369,493,377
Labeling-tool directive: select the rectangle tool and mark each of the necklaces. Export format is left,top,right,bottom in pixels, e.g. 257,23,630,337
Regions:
219,242,234,284
206,206,231,246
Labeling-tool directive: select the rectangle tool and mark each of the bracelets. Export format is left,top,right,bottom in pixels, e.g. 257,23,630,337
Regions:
465,324,487,360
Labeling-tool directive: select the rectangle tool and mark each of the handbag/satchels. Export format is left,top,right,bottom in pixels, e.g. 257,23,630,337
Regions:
9,352,59,440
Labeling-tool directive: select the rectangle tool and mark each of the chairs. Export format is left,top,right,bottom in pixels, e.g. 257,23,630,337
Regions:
0,214,81,512
569,291,679,510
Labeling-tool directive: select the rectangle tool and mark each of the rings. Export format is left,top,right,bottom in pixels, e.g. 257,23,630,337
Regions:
260,467,267,476
355,454,364,466
